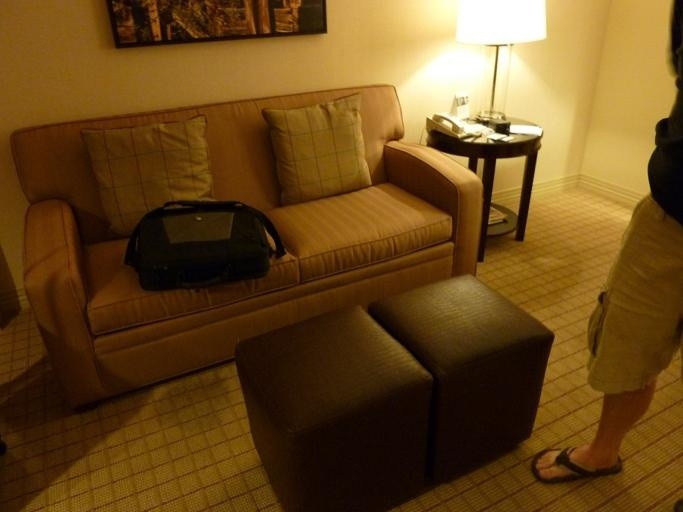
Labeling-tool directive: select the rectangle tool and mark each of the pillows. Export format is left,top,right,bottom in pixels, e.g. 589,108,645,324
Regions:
261,92,371,207
78,115,214,237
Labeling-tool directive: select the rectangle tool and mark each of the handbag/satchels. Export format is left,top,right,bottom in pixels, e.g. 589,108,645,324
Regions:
123,197,287,292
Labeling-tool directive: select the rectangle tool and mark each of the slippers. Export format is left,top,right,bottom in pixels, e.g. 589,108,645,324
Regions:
529,447,625,483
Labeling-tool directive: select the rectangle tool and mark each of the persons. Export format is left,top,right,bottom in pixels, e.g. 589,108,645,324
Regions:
531,0,683,511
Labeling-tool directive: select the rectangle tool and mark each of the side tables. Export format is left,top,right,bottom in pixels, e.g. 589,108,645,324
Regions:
425,115,543,263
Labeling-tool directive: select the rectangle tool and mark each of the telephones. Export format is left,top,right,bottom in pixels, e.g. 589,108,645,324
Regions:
426,112,480,138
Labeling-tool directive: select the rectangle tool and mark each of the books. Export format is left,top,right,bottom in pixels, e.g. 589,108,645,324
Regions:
488,206,506,224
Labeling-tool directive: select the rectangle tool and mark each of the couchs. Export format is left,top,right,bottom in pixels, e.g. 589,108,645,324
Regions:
8,83,484,414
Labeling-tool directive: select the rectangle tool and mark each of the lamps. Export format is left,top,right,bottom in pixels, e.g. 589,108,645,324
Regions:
453,0,546,124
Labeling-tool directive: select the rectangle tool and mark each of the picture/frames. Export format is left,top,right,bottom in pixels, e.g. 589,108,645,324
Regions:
105,0,328,48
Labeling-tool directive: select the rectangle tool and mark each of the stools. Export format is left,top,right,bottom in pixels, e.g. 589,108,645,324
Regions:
233,305,435,512
369,273,556,469
